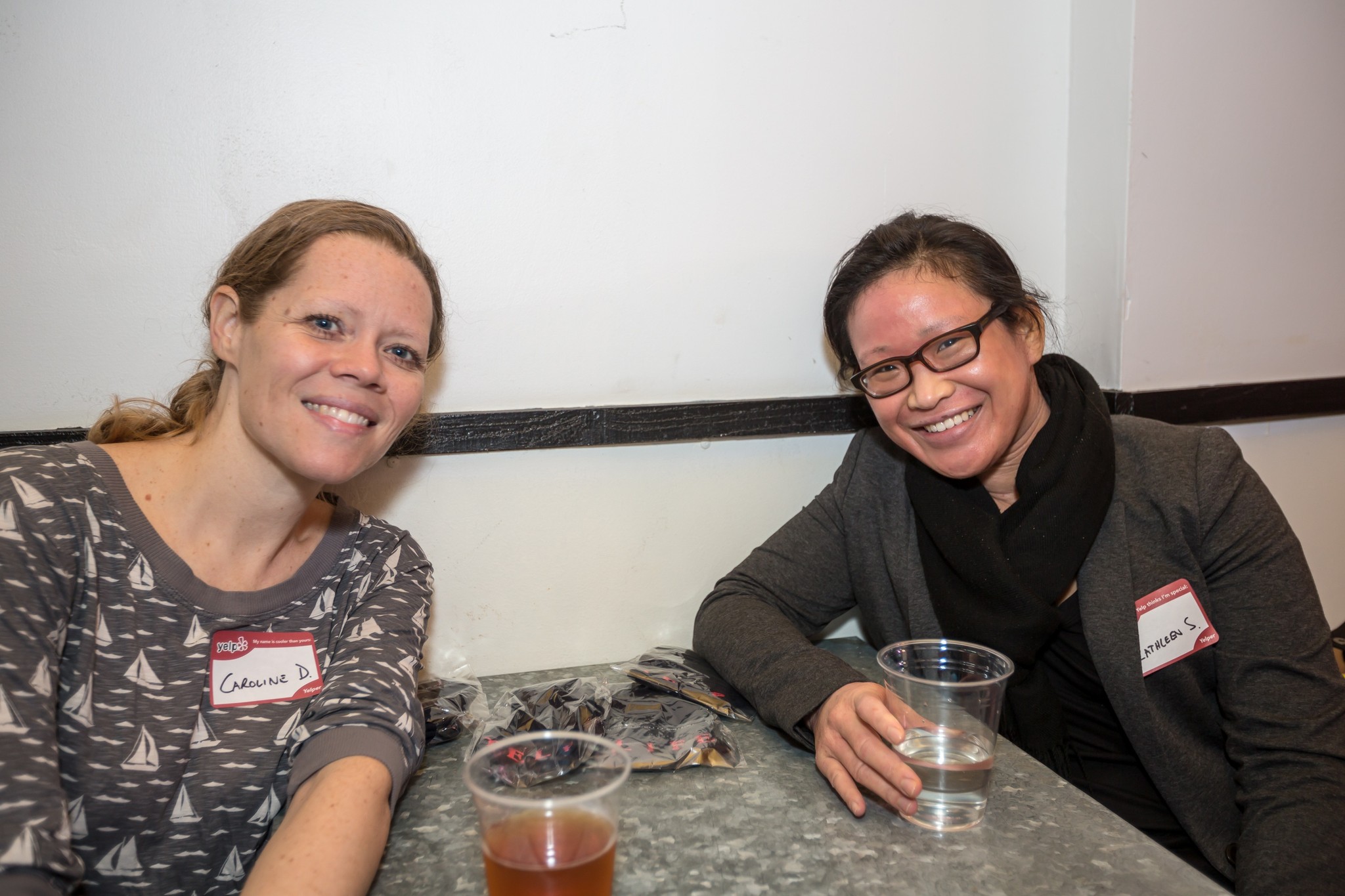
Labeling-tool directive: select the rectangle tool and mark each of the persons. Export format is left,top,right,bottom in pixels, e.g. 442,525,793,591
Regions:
1,200,447,894
694,209,1345,895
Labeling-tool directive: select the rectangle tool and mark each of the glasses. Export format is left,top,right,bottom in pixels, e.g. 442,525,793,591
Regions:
849,302,1012,399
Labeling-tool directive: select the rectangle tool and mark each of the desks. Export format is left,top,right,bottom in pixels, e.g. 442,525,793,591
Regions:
347,634,1232,896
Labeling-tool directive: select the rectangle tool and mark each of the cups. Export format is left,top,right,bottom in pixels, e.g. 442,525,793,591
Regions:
463,730,632,896
877,637,1014,832
